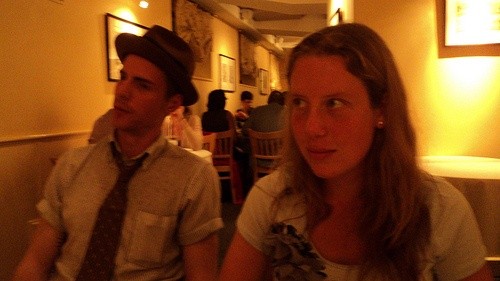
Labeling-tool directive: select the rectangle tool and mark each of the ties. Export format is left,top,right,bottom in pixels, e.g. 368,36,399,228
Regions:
74,141,149,281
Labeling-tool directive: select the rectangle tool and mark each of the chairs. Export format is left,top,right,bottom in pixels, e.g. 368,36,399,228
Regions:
203,131,243,204
248,127,286,185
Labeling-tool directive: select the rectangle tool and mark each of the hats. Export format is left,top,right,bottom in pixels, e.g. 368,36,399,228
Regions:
115,24,199,107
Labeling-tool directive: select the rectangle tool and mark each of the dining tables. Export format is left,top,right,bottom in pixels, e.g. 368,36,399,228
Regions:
415,155,500,257
182,147,213,163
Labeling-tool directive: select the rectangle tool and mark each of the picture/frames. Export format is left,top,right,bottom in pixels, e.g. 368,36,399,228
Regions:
443,0,500,47
106,13,151,82
259,68,269,95
219,54,236,92
329,8,341,27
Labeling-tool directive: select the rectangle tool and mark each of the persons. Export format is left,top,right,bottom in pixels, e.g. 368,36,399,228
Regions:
13,25,225,281
232,91,256,123
242,91,286,162
218,23,494,281
161,105,203,152
201,90,248,196
